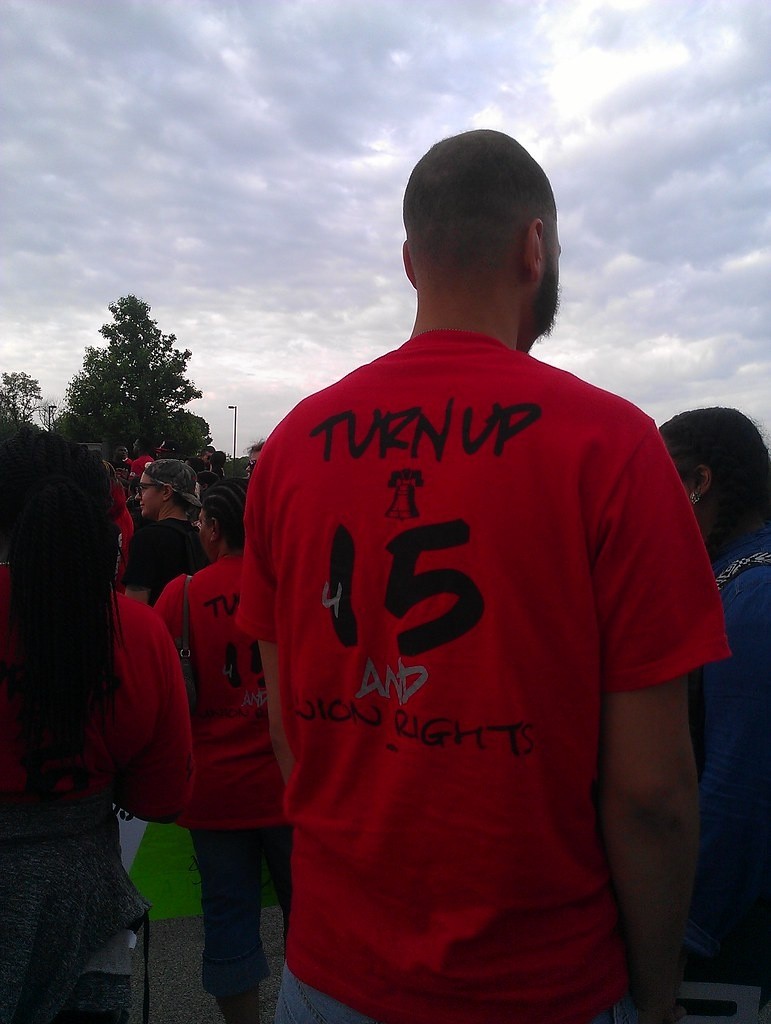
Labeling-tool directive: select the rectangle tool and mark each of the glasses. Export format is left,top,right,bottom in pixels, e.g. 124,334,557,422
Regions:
137,483,158,491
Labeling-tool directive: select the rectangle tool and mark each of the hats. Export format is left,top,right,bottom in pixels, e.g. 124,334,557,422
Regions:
153,440,178,453
144,459,203,507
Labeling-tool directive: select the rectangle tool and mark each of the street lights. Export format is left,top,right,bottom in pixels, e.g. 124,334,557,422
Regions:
229,406,236,478
49,406,56,431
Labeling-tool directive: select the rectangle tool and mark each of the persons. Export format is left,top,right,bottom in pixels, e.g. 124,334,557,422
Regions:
650,404,771,1024
0,421,307,1022
234,128,732,1023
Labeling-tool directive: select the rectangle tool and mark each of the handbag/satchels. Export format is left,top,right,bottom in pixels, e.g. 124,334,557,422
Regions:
179,575,198,715
